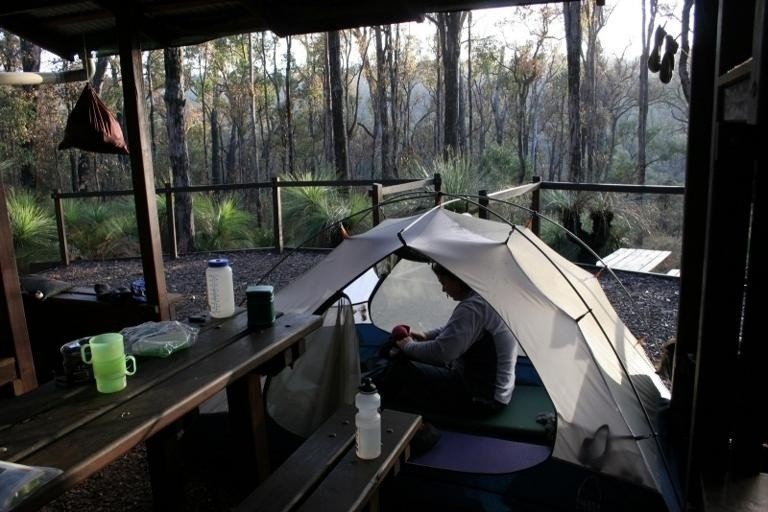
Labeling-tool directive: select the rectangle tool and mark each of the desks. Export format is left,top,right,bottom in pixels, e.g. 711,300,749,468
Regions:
0,307,323,512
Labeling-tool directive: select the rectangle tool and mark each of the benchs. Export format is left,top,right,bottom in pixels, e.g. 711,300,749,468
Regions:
23,288,183,321
235,404,424,512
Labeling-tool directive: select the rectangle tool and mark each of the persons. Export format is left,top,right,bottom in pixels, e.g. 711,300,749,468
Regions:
386,264,518,415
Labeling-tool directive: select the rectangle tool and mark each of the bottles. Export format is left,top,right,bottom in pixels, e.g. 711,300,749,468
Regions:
205,258,235,319
354,377,382,461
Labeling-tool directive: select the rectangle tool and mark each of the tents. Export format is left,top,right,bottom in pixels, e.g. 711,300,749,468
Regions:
197,191,685,512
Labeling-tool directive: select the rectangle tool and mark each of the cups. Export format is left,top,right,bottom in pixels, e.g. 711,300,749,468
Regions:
81,333,137,394
